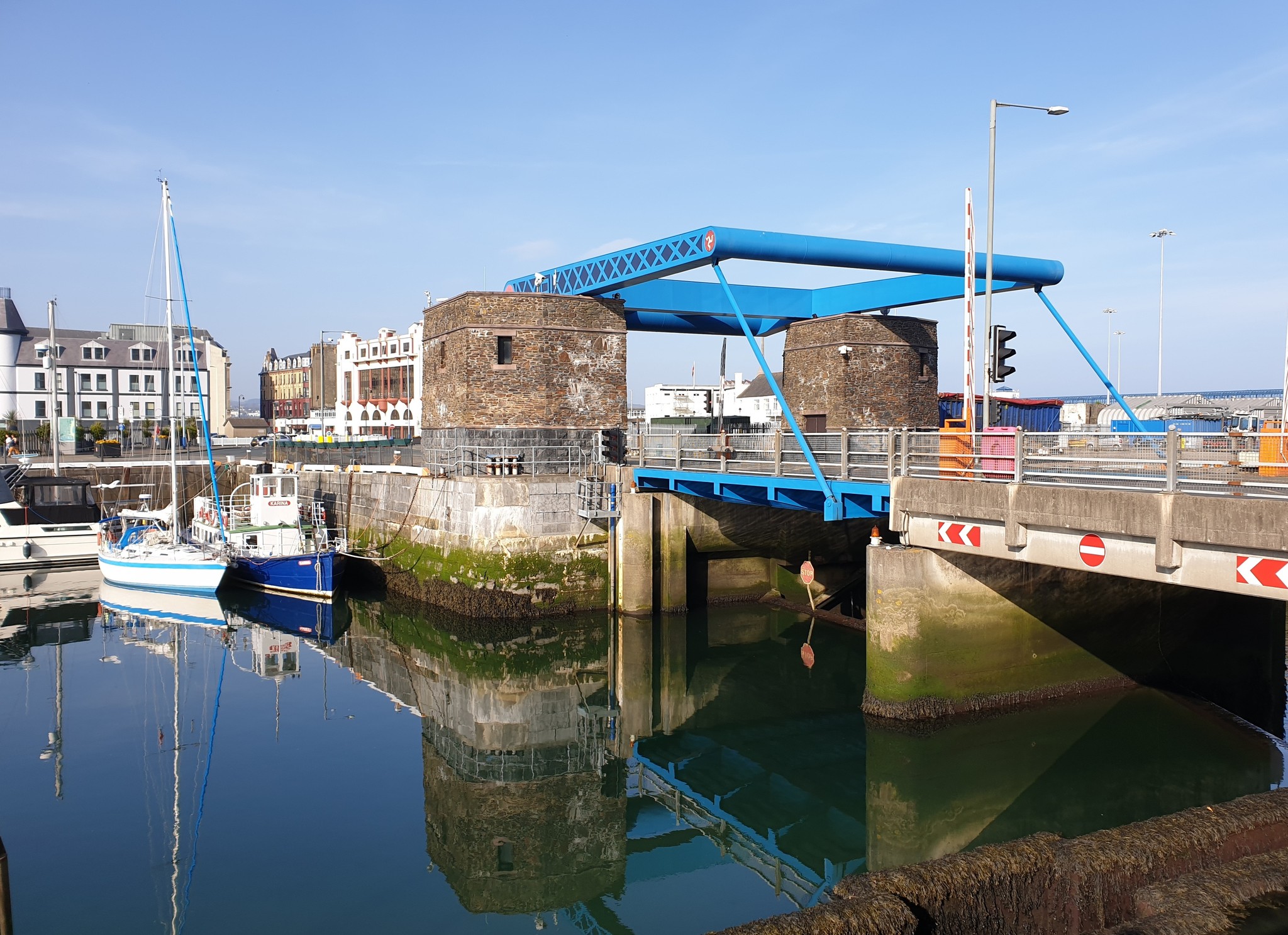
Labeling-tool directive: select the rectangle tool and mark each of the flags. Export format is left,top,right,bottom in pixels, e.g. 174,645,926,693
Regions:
692,365,694,377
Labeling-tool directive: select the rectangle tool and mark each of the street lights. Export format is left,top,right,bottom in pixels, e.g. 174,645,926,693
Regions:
365,387,373,435
399,353,411,439
345,375,349,431
980,101,1069,427
1101,307,1118,405
297,392,303,434
1113,330,1126,394
319,330,352,436
238,395,245,417
1147,227,1177,396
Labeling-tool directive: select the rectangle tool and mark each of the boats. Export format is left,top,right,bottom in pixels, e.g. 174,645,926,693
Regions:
180,402,353,605
215,583,354,746
0,587,102,801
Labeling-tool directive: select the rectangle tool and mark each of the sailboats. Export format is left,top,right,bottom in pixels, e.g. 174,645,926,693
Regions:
97,580,242,935
97,167,231,593
0,297,117,562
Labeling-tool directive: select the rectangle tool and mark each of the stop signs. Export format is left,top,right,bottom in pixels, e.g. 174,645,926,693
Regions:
800,643,815,669
390,424,395,429
1078,533,1107,567
801,561,813,585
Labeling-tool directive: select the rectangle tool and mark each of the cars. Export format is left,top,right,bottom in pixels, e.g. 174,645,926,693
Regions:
370,433,383,436
251,431,293,447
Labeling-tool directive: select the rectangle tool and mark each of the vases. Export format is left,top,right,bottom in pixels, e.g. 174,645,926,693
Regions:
150,438,170,449
94,443,121,457
413,438,421,445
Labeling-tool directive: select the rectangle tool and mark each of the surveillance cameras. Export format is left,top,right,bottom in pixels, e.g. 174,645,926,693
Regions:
840,349,847,356
424,292,429,295
328,338,333,342
535,272,546,280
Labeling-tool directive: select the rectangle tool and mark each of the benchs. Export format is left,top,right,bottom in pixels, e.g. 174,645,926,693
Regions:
269,436,412,448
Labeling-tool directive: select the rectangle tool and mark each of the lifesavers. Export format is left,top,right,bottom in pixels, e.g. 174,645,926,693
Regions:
198,508,203,519
97,531,101,545
219,512,228,531
204,509,211,525
319,506,326,522
298,502,304,521
211,511,217,527
308,505,314,522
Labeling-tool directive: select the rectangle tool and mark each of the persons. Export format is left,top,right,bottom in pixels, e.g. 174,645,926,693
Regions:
2,434,21,458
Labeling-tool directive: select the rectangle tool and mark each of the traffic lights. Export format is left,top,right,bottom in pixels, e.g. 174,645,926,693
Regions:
703,390,712,413
601,427,620,463
991,399,1002,425
993,323,1016,383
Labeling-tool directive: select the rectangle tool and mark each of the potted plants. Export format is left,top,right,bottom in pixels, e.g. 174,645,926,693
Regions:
120,428,132,448
35,421,50,455
143,431,153,446
76,426,86,452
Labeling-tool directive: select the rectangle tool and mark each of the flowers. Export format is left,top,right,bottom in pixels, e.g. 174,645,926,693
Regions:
413,435,421,438
96,440,119,444
158,435,168,438
